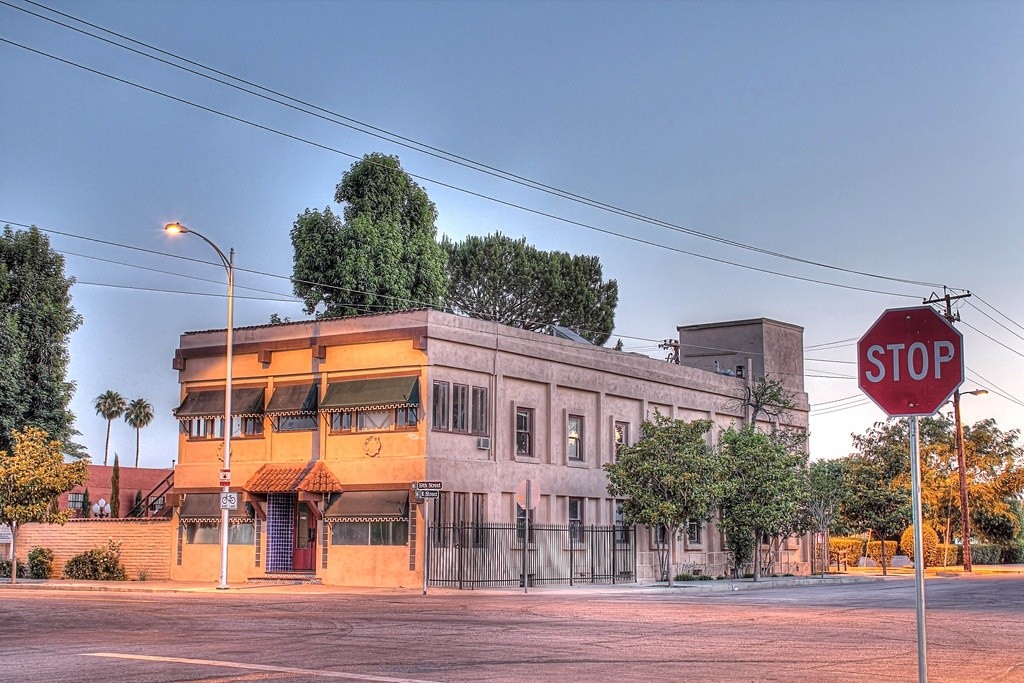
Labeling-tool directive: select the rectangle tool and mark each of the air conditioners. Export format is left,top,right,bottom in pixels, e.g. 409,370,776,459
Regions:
477,438,492,450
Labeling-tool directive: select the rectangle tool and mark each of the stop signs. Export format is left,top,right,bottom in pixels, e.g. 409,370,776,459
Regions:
856,306,967,417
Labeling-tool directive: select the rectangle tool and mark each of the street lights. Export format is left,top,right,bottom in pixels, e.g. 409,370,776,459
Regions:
953,389,989,572
163,222,234,589
